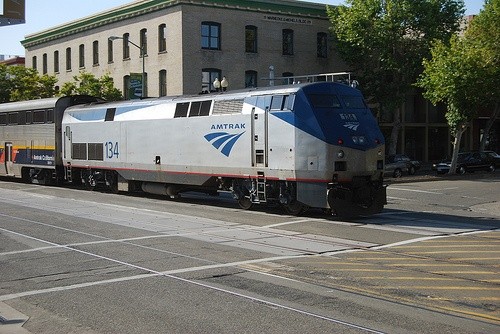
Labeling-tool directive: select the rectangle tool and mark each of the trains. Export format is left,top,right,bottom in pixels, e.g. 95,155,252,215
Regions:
0,76,390,221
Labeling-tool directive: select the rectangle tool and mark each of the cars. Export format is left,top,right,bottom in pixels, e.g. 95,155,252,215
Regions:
382,152,426,179
432,149,500,175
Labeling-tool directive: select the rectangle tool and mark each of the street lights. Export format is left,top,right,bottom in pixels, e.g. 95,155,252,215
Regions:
108,35,147,98
